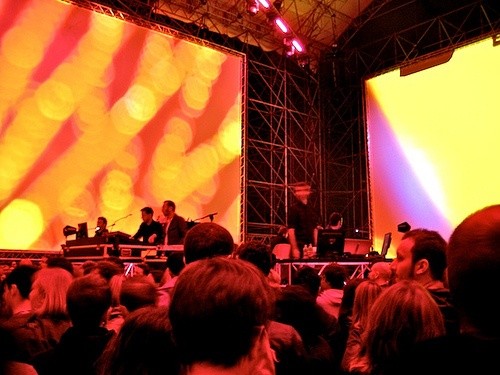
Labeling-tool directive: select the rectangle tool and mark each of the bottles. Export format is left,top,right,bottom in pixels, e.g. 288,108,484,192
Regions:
303,243,313,259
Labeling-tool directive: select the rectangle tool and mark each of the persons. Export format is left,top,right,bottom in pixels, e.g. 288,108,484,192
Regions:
389,228,460,335
18,267,74,361
94,217,108,237
379,205,500,375
359,281,446,375
106,305,182,375
0,200,500,375
160,200,186,245
132,206,164,246
44,275,114,375
286,182,325,260
324,211,343,230
168,257,276,375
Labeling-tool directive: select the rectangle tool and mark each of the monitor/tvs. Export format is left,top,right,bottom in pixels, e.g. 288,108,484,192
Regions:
76,222,88,238
381,232,391,261
318,234,345,262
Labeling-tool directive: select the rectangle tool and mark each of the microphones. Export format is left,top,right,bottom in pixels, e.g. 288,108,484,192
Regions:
110,214,132,232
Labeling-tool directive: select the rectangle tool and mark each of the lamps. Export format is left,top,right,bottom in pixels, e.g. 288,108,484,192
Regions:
247,1,303,57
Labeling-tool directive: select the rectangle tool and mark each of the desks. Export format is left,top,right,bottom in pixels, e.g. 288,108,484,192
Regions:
274,260,374,286
60,244,171,268
